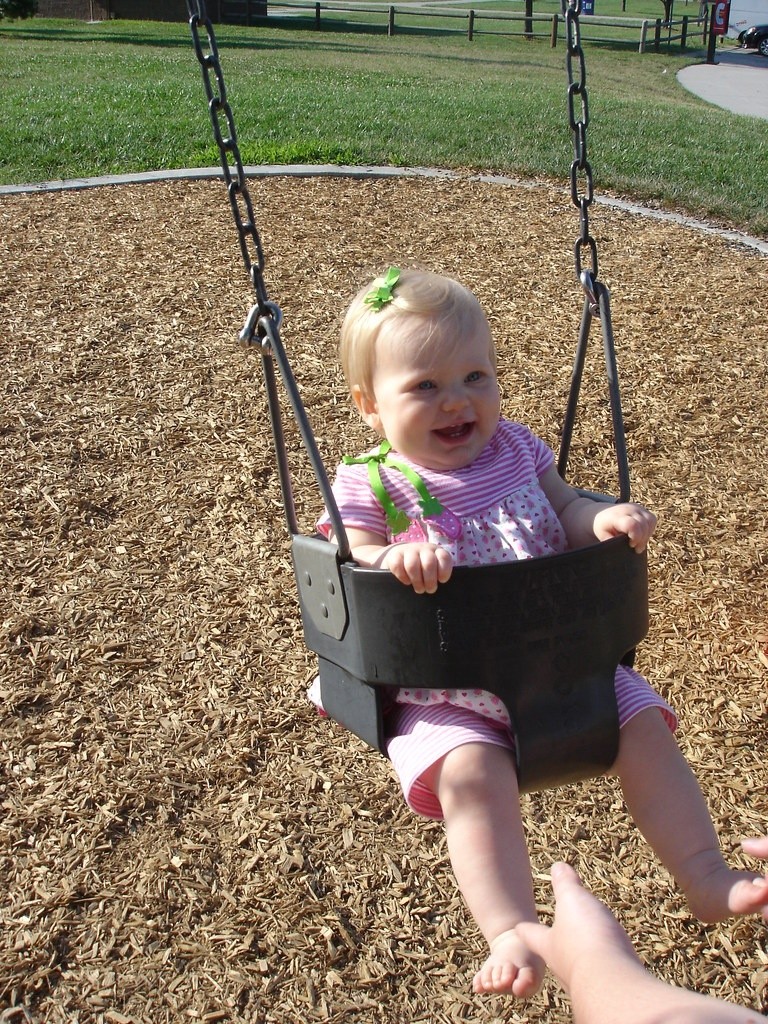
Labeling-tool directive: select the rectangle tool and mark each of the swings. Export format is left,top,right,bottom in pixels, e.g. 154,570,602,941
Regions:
178,1,652,797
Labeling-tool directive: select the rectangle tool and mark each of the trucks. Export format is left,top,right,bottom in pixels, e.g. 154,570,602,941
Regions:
727,0,768,46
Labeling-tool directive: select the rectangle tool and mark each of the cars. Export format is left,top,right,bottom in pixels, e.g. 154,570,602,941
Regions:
743,23,768,56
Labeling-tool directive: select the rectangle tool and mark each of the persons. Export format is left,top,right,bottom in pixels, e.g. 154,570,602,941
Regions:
514,835,768,1024
308,267,767,999
